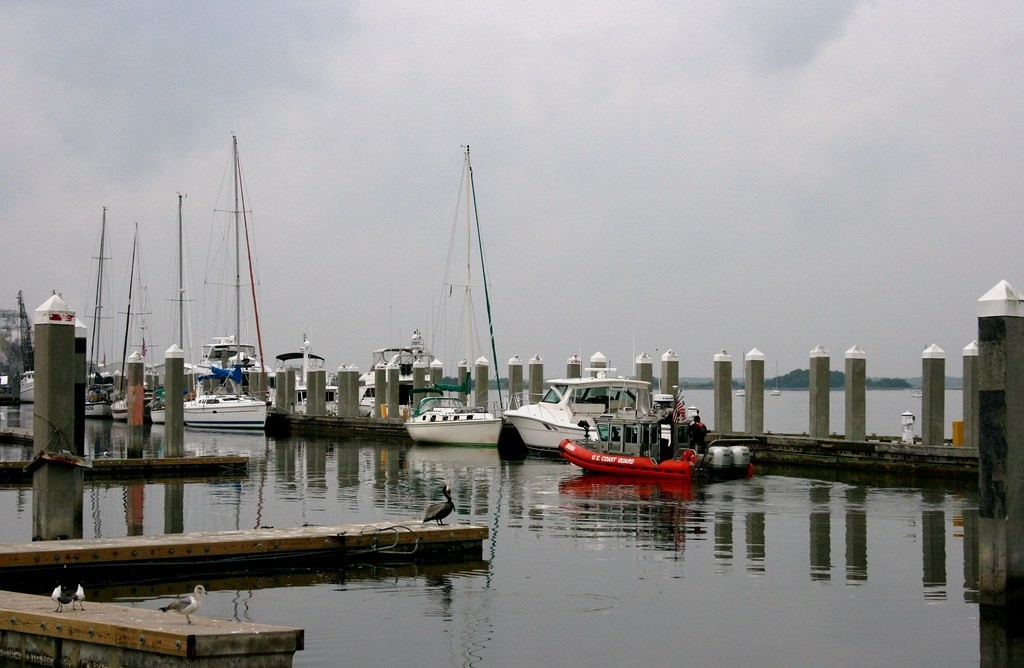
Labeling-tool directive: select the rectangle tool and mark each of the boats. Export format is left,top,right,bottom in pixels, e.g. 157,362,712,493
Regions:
557,379,760,482
19,371,34,402
267,334,338,414
357,348,435,416
502,377,693,453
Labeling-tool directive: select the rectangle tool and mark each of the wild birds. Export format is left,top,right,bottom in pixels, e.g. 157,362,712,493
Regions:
155,583,209,626
103,448,112,460
50,574,88,613
421,485,457,526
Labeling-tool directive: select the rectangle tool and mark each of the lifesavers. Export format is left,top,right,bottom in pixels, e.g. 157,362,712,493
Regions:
681,450,696,467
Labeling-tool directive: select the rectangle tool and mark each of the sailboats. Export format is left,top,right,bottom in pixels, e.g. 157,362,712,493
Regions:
85,206,113,419
183,136,274,435
403,145,508,446
111,222,151,421
151,194,195,424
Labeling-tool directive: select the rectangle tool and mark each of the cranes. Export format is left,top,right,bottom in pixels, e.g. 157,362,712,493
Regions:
16,290,34,372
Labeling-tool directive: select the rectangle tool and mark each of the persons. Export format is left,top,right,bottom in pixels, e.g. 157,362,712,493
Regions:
689,415,707,451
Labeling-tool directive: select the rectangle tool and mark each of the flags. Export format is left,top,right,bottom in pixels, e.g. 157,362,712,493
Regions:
672,388,686,424
